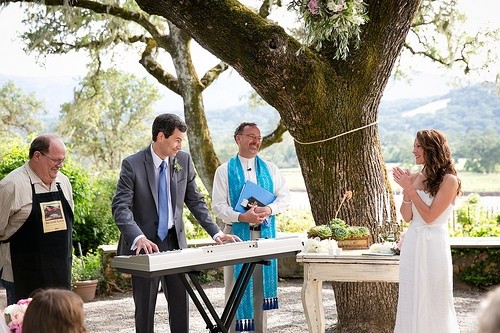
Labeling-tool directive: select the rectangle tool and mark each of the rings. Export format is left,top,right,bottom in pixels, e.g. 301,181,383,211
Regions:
398,176,400,179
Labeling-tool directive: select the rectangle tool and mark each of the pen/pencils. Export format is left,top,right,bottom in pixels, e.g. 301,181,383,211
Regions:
248,204,253,207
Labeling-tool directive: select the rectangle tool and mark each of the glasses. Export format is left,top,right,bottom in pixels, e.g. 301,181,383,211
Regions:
41,152,64,165
238,133,261,141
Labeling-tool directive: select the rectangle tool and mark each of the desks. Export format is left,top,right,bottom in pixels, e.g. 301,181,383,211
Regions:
296,250,400,333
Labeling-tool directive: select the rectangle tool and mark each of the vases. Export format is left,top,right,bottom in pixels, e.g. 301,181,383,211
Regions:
322,235,372,250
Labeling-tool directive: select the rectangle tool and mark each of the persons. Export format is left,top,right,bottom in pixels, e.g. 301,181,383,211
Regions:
22,289,86,333
111,114,243,333
0,135,75,308
392,129,463,333
211,122,291,333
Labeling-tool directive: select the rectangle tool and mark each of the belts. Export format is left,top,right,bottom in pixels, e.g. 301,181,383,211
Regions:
227,224,261,231
168,227,175,234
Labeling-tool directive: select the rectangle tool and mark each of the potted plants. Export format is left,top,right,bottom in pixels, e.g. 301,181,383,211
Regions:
72,246,102,302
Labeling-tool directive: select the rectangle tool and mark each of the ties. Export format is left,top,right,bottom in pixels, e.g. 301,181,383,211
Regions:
158,161,168,241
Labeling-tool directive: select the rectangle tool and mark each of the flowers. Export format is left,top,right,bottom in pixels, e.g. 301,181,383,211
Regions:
308,191,370,240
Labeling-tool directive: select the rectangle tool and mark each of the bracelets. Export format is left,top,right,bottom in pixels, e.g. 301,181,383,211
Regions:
402,200,412,204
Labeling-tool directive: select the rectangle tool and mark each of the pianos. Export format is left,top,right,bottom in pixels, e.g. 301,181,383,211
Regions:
111,235,304,278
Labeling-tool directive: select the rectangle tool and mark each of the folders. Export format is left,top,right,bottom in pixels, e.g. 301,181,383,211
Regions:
234,180,276,213
